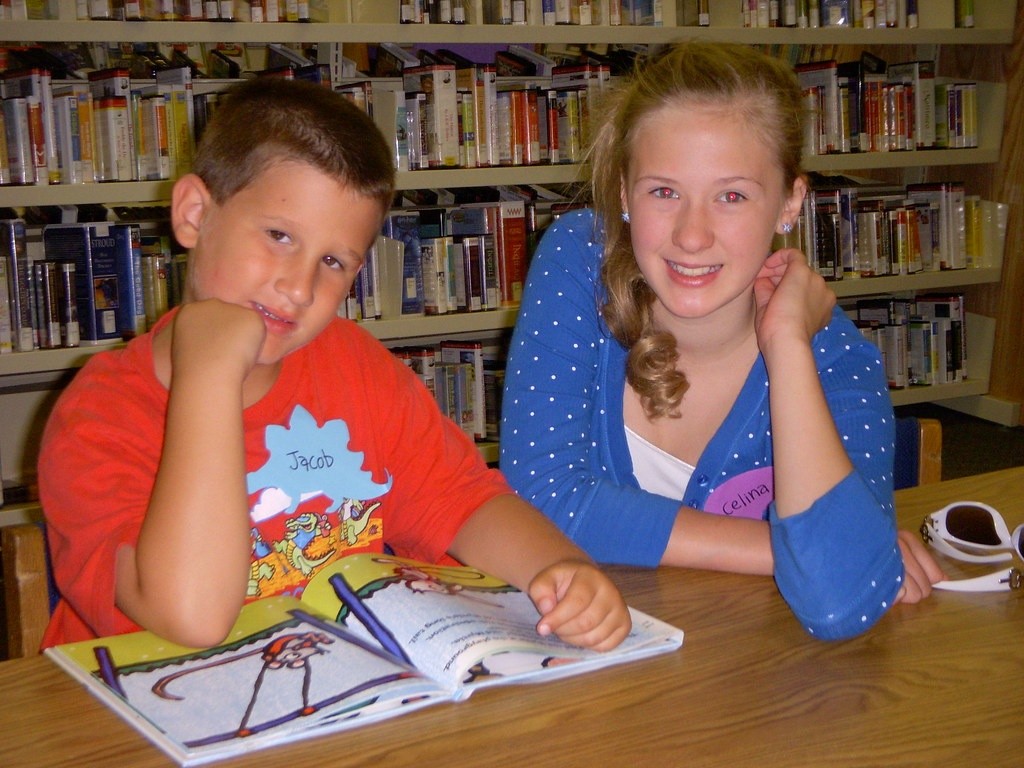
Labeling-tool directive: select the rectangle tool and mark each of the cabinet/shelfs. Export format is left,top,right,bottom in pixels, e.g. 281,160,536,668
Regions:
0,0,1024,483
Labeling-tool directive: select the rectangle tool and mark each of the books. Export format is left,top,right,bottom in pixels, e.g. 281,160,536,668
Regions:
43,551,684,768
0,0,1008,445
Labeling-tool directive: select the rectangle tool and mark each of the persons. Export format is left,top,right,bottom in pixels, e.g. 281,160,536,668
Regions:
502,39,947,646
36,73,633,654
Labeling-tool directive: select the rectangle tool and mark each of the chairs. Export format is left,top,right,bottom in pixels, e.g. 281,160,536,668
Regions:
895,417,942,488
3,524,50,659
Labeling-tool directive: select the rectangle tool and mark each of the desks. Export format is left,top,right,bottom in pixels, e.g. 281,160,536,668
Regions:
0,456,1024,768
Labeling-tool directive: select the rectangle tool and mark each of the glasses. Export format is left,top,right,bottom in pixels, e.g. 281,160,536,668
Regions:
919,501,1024,592
